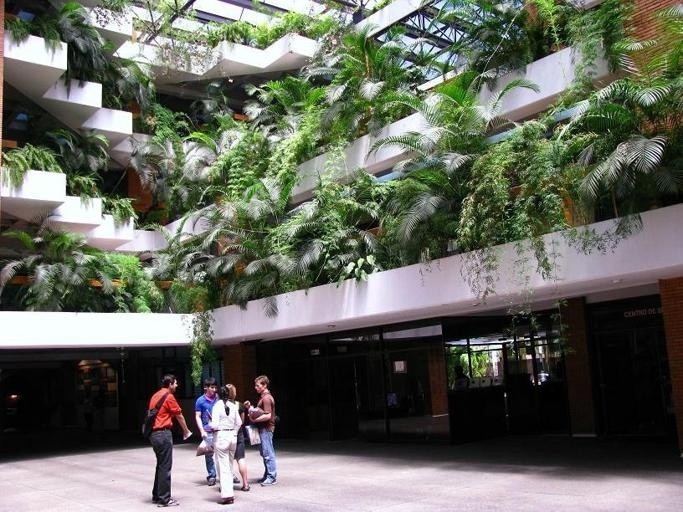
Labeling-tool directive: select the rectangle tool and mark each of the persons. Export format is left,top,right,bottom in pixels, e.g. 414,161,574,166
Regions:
207,385,243,505
145,373,193,508
243,373,279,486
451,365,470,390
223,383,250,492
194,376,240,487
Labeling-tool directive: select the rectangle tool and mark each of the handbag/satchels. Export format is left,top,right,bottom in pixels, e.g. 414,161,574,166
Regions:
245,425,262,446
142,408,158,439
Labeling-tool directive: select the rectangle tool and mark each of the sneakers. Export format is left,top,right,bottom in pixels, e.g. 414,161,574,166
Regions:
208,478,216,486
216,497,234,504
152,497,179,507
258,475,279,486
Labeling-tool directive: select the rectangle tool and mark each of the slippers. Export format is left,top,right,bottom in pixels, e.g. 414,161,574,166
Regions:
233,477,250,491
